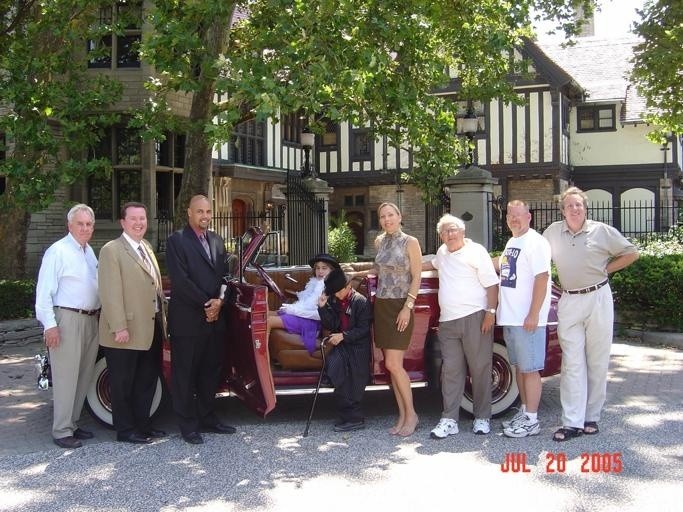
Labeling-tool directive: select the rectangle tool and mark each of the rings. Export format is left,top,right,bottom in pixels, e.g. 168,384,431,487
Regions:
404,321,407,325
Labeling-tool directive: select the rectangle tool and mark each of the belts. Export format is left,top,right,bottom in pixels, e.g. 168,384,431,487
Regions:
53,305,102,316
564,279,608,294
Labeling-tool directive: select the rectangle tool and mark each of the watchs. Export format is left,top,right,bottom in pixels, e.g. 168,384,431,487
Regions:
405,302,415,309
485,307,496,314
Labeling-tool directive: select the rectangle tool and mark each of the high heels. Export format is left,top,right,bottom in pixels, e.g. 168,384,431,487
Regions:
398,417,419,437
387,424,400,435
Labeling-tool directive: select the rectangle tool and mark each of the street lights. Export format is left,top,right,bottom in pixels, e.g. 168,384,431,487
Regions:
461,109,478,166
657,129,672,229
298,126,316,178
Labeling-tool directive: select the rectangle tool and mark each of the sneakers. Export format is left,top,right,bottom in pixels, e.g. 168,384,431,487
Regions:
430,418,459,440
472,417,491,435
503,412,541,438
501,408,527,429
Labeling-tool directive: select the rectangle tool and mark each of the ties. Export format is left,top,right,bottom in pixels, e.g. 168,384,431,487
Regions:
200,232,211,261
138,246,151,273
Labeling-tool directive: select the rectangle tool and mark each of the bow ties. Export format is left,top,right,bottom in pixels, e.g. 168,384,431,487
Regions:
340,302,346,306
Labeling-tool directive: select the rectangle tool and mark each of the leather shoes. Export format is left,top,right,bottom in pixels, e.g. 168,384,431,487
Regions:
73,428,94,439
116,430,153,444
198,423,236,434
53,436,82,448
181,429,204,444
333,421,365,432
145,429,166,438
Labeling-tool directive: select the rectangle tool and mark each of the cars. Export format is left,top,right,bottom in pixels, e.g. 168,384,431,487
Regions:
36,225,561,432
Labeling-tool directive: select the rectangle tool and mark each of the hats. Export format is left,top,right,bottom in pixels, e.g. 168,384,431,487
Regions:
308,253,340,270
322,269,352,296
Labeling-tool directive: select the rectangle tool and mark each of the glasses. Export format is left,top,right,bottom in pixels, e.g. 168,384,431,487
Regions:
439,228,458,236
506,214,528,219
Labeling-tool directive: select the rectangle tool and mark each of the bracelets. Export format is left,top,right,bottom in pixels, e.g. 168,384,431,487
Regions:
406,292,417,299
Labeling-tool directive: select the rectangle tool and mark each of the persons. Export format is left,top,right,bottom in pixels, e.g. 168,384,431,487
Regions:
422,213,500,421
493,199,551,421
267,254,341,357
119,202,162,443
165,195,236,445
542,187,639,442
318,269,372,432
346,203,422,437
35,202,100,449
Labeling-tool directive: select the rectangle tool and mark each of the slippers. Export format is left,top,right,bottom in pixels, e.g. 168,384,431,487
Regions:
553,428,584,442
583,422,599,436
